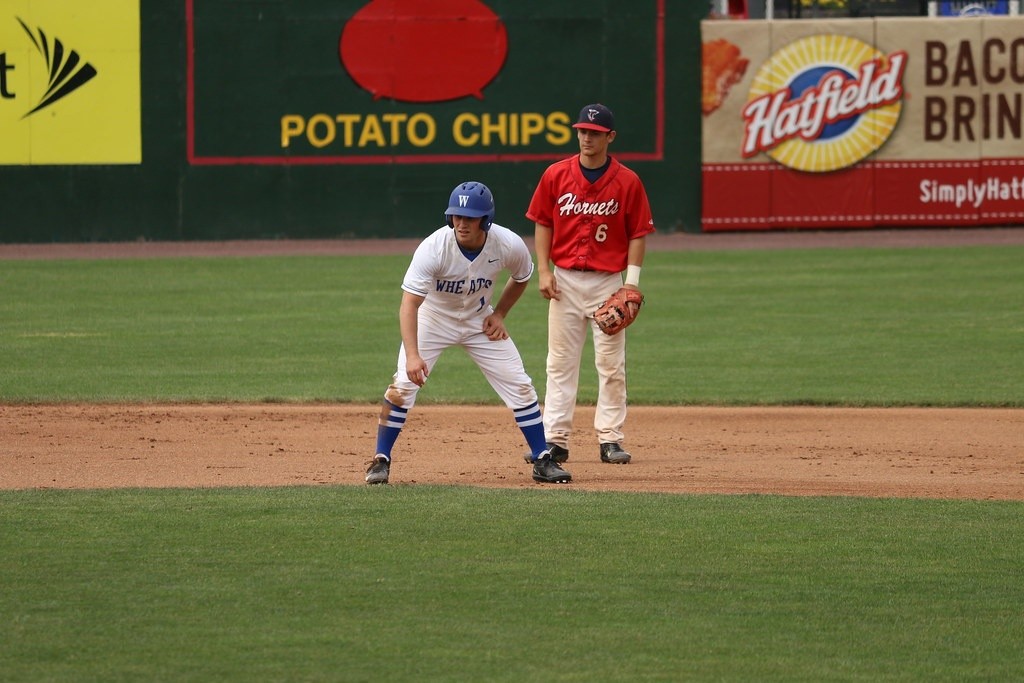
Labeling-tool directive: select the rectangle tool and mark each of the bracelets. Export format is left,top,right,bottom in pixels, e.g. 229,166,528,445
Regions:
625,264,641,288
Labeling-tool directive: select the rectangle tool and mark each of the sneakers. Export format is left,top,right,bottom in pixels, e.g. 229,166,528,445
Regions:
523,442,568,465
532,453,571,484
364,457,390,484
600,443,631,465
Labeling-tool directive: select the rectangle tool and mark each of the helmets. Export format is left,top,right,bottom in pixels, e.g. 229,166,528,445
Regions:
444,182,495,231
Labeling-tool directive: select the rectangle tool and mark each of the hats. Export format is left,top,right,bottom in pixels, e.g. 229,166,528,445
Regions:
572,103,616,132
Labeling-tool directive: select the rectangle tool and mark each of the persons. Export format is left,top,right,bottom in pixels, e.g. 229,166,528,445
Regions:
523,103,656,464
364,181,572,484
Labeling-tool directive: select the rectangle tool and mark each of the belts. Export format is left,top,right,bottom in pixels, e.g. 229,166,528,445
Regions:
573,268,595,272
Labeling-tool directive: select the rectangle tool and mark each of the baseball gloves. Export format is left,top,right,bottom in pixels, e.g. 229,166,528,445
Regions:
592,287,646,337
